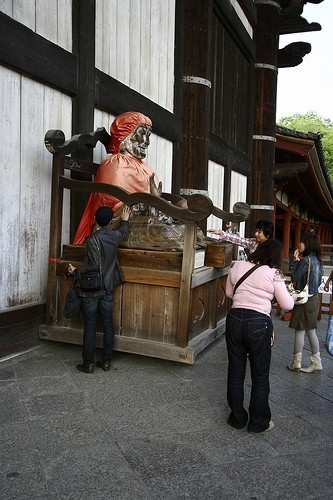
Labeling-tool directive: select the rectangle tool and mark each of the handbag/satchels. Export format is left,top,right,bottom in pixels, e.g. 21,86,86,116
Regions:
80,267,104,292
61,269,83,319
291,283,309,306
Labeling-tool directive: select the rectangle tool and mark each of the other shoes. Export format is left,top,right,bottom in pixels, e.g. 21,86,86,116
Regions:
97,360,111,371
268,421,274,431
77,363,96,374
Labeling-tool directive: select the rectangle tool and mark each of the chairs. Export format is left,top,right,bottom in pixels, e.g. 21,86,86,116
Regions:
38,126,251,366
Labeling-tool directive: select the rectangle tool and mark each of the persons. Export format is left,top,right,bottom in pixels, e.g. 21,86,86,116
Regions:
244,220,282,347
239,250,248,261
207,229,259,261
225,239,294,434
68,206,132,373
74,112,206,251
287,232,323,375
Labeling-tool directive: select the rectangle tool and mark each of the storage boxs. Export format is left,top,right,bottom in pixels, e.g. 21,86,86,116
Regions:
205,243,232,267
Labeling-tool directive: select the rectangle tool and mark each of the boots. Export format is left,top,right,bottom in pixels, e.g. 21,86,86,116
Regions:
287,351,303,372
299,352,324,374
